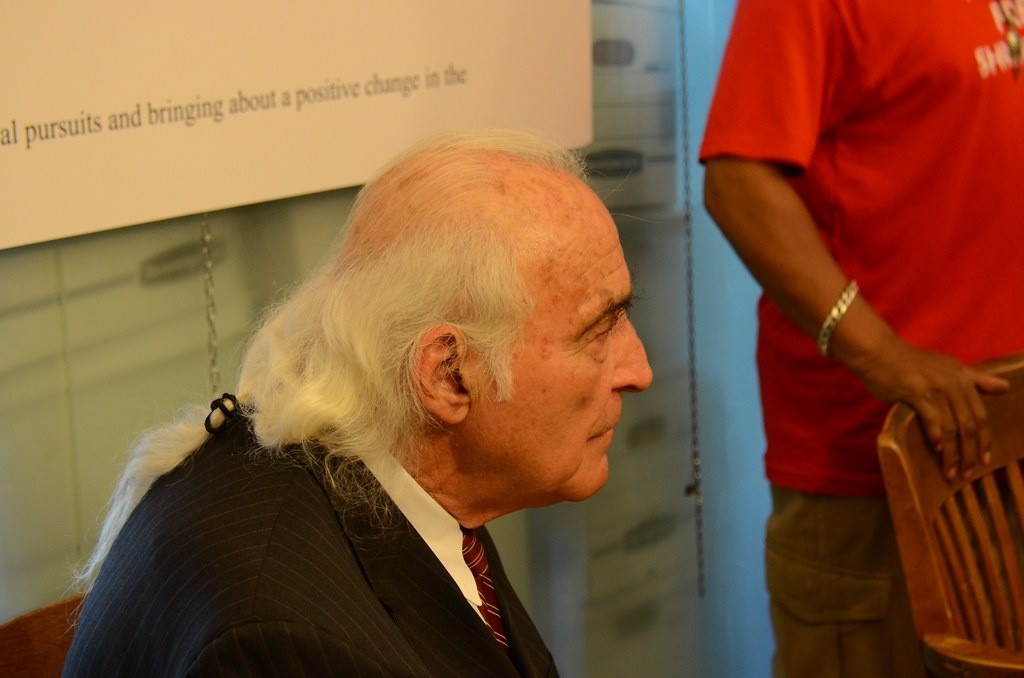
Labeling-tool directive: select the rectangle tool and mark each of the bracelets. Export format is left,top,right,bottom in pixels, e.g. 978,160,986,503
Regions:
815,280,857,360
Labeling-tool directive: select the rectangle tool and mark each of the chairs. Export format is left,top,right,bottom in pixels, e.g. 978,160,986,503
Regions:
877,352,1024,678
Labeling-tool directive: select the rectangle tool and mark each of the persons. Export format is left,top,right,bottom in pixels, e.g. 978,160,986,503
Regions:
60,130,653,678
697,0,1024,678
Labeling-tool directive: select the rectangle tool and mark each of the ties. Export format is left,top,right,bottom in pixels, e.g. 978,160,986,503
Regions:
462,529,510,653
